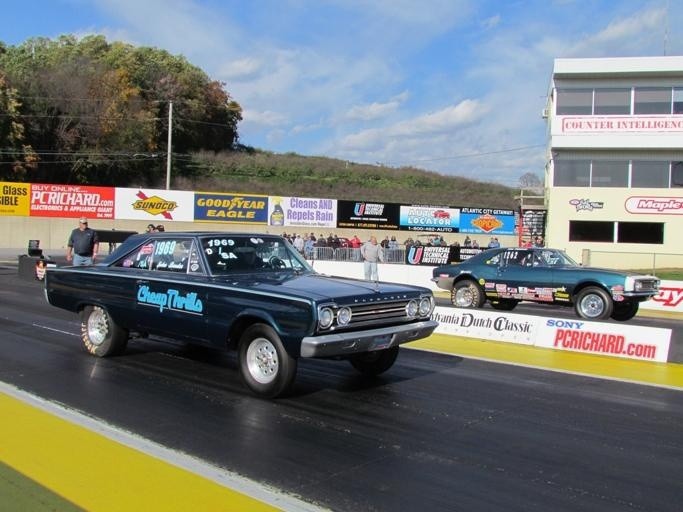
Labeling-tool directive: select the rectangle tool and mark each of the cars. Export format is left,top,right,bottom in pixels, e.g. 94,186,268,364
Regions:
43,232,439,397
430,247,663,324
328,237,364,258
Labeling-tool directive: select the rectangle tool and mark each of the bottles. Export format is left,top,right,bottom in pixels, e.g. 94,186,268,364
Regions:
270,200,284,225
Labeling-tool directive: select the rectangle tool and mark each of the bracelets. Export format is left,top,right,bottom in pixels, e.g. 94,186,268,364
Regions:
92,252,96,255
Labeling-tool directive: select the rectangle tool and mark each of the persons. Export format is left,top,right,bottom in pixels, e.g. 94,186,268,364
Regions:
380,235,544,262
155,225,164,233
107,227,116,255
147,224,156,233
349,235,361,262
276,233,352,261
360,235,383,281
227,245,267,270
65,216,99,268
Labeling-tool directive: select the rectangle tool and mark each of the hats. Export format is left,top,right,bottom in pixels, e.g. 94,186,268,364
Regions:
79,216,89,224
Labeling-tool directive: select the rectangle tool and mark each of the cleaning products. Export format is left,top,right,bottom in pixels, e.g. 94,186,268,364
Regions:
270,197,284,226
